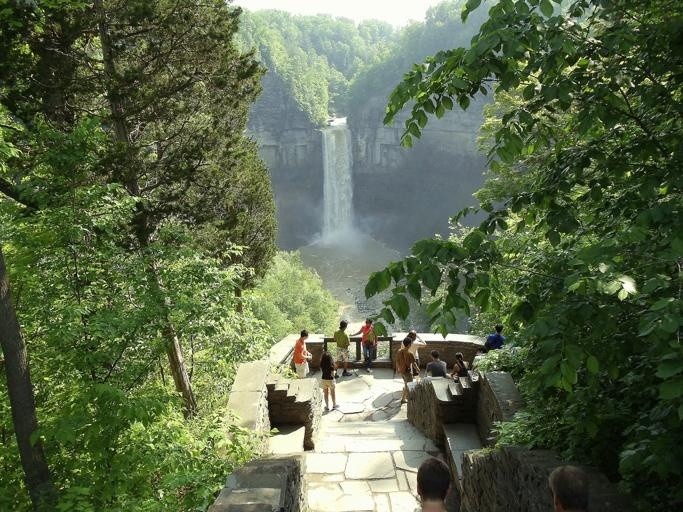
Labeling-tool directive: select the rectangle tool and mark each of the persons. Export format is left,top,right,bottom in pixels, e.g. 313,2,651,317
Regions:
400,330,427,378
547,464,589,511
349,317,378,373
396,336,421,404
416,456,452,512
476,347,487,354
319,352,339,410
484,323,506,349
424,349,451,378
333,319,352,378
294,329,312,378
451,351,470,377
